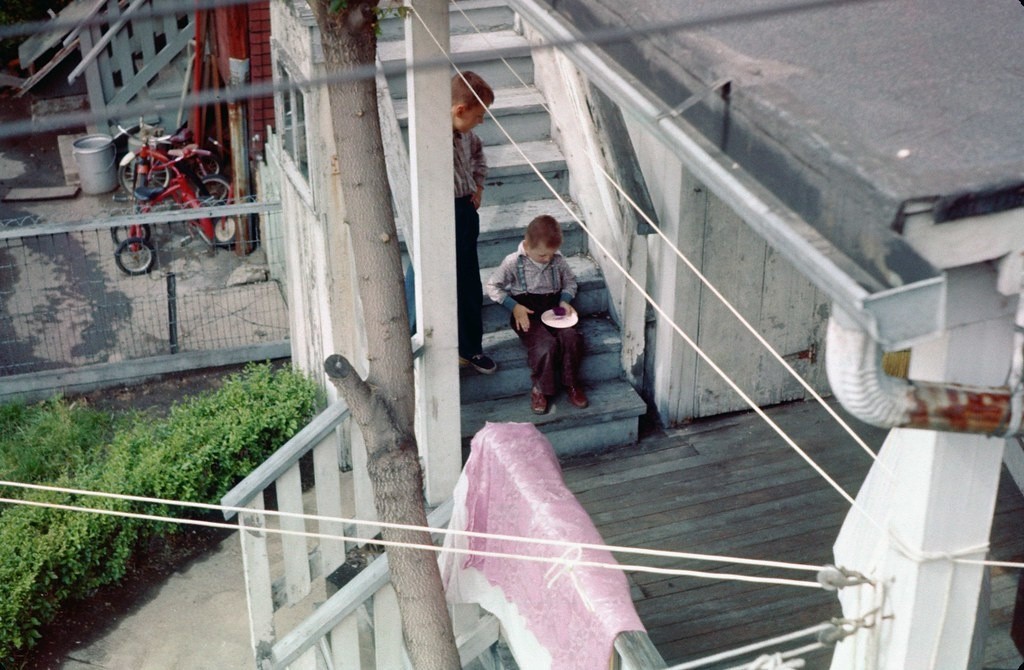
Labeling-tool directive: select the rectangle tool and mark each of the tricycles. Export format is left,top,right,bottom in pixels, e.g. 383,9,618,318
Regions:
109,115,238,276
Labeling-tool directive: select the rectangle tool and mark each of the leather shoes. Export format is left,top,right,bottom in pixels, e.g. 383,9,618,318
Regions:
566,385,588,408
531,386,547,414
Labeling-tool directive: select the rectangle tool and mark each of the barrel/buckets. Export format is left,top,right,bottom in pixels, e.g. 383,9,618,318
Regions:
72,133,119,195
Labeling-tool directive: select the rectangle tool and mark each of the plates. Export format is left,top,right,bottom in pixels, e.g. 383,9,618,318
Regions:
541,308,579,328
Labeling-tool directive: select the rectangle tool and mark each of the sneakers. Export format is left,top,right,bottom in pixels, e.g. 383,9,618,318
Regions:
459,353,497,374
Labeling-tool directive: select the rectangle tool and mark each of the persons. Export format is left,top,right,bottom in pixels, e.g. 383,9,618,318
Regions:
451,68,498,375
488,215,589,413
